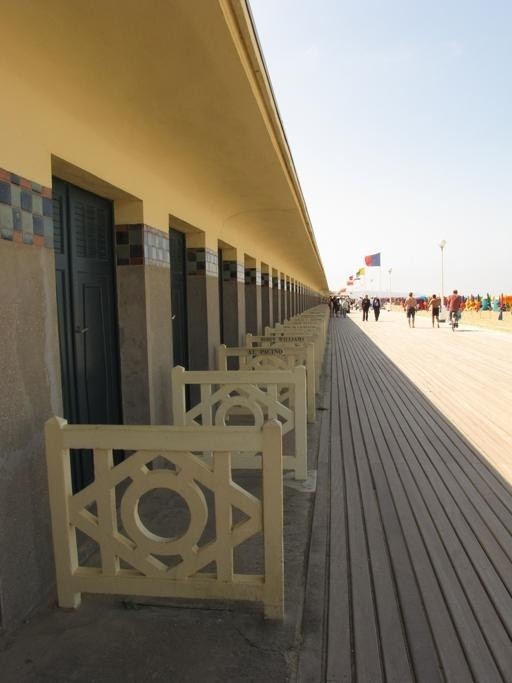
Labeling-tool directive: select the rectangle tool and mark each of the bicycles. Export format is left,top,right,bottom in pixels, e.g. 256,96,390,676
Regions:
447,308,459,330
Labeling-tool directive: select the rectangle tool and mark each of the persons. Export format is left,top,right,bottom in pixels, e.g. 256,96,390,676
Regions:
324,289,512,331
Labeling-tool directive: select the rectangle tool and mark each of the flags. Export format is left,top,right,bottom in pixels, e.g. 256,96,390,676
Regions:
334,252,380,294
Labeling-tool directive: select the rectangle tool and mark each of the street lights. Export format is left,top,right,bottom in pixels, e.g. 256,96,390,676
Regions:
387,267,393,304
371,279,374,296
438,239,447,296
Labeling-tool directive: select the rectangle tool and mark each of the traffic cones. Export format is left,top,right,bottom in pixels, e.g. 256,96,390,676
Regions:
498,310,502,320
